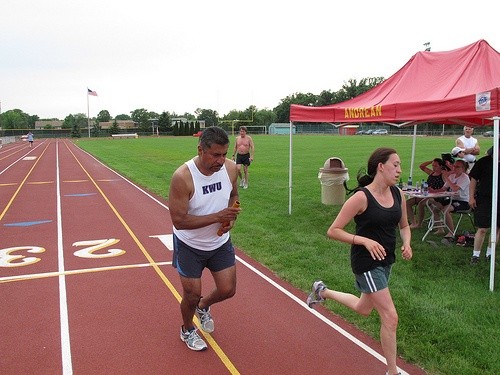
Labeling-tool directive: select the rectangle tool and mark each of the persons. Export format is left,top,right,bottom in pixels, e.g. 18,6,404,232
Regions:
232,126,254,189
406,156,470,239
168,126,240,351
306,147,412,375
468,145,500,263
27,131,34,148
452,147,466,163
457,126,480,172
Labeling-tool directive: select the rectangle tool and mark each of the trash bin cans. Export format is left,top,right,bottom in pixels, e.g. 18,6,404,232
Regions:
319,157,348,206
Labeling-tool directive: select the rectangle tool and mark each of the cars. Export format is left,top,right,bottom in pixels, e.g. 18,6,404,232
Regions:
357,129,388,135
483,131,493,137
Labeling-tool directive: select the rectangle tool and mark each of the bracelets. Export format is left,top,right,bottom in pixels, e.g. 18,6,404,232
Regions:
233,155,234,156
353,235,355,243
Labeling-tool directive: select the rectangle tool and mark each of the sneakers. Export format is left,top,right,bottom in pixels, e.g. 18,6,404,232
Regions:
306,279,326,308
386,371,402,375
195,296,215,334
180,323,208,351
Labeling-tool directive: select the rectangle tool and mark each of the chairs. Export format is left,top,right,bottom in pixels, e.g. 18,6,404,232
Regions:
453,207,477,239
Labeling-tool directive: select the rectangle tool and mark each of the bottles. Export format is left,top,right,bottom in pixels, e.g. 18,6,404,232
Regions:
407,176,412,190
423,182,428,196
399,177,403,189
421,178,425,192
415,181,419,192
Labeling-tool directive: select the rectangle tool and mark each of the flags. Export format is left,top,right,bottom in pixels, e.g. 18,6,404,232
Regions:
88,89,97,96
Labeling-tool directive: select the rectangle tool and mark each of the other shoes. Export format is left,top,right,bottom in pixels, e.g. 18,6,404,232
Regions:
239,178,249,189
484,254,492,263
470,256,482,266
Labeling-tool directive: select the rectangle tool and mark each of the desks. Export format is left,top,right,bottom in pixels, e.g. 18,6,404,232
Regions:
395,184,458,242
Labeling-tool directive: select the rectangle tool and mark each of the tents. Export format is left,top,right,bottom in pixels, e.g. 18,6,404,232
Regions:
289,38,500,291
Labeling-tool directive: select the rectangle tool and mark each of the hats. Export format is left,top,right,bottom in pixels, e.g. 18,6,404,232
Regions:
450,146,466,156
